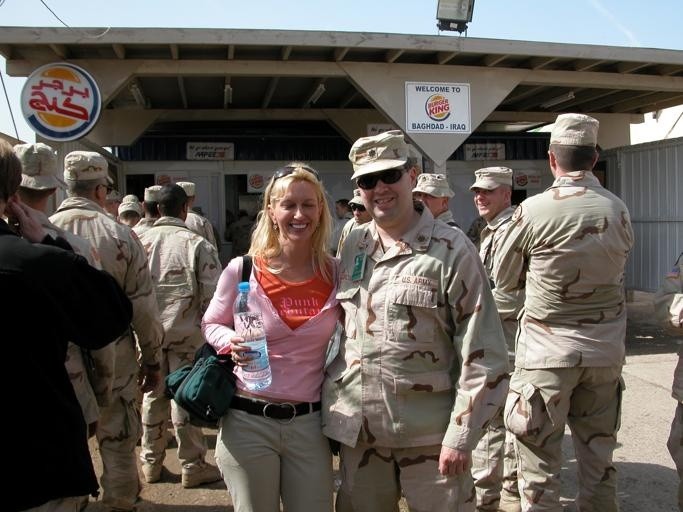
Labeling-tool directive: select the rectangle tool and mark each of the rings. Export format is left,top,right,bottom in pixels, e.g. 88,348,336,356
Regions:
231,353,236,360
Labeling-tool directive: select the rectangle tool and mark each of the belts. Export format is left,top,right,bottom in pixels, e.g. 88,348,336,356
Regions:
229,395,321,423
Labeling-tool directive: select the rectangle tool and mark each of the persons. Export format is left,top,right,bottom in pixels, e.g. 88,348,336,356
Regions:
652,237,683,512
317,132,509,512
332,187,373,269
137,184,226,486
411,173,466,251
170,180,218,277
224,207,262,257
492,113,634,512
200,164,347,512
0,136,131,512
42,149,165,512
5,144,116,508
327,197,350,249
116,195,144,228
467,164,532,512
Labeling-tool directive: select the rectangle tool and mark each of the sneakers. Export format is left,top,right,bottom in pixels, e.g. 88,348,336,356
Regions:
182,464,220,487
142,464,162,482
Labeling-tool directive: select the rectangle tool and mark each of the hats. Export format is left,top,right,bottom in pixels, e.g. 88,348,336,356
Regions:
144,186,163,202
470,167,513,189
12,143,67,189
118,202,140,215
123,195,139,202
177,182,196,196
550,113,599,147
413,174,454,198
349,130,408,180
348,189,365,205
64,150,114,185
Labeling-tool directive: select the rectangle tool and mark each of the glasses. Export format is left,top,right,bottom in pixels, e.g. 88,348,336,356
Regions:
356,170,401,190
274,166,320,183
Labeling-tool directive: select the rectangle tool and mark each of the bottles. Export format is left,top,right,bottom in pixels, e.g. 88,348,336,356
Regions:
233,283,271,390
